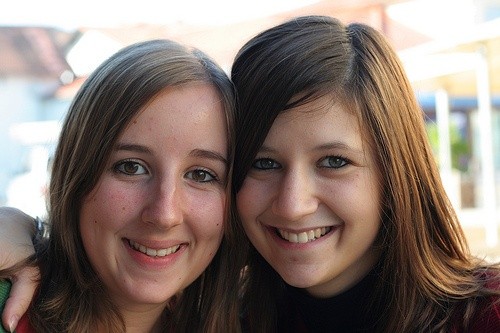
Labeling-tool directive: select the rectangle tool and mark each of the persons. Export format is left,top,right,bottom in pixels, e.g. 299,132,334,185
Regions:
0,13,500,333
1,38,237,332
